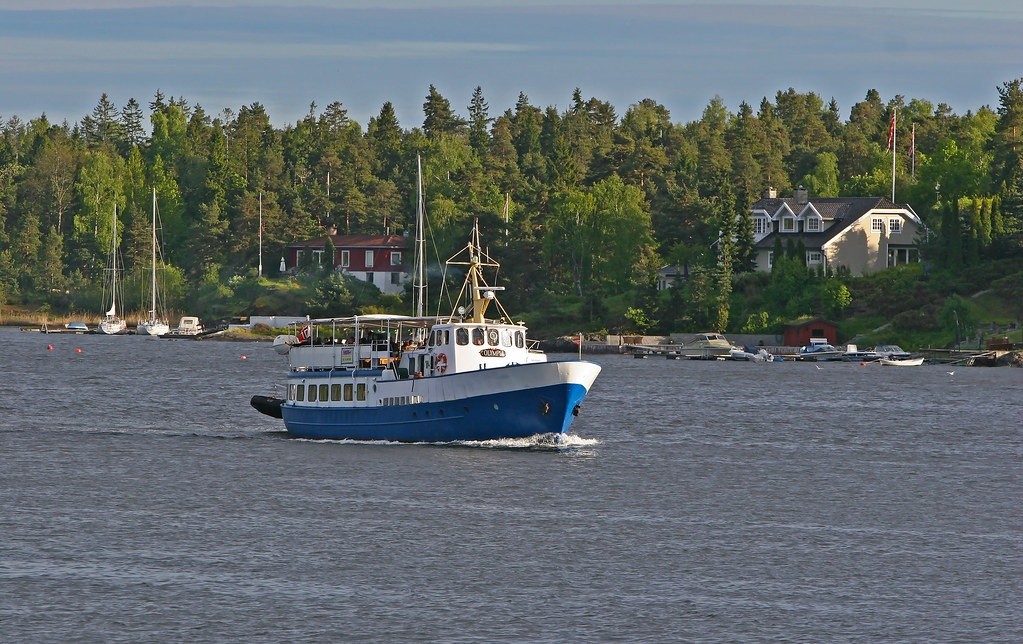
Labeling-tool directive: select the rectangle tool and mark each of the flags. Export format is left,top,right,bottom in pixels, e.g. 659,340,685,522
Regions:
908,127,913,156
886,110,895,154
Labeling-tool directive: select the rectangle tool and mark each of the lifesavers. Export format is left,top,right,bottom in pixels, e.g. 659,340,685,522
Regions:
435,353,448,373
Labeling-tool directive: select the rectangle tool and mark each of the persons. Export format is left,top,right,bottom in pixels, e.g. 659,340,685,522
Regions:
307,335,355,345
401,340,419,356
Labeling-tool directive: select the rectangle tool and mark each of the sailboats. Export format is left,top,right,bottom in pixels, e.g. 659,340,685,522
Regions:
67,187,205,335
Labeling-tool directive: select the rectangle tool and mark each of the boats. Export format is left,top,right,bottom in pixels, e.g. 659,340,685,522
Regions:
669,332,926,367
275,150,603,452
